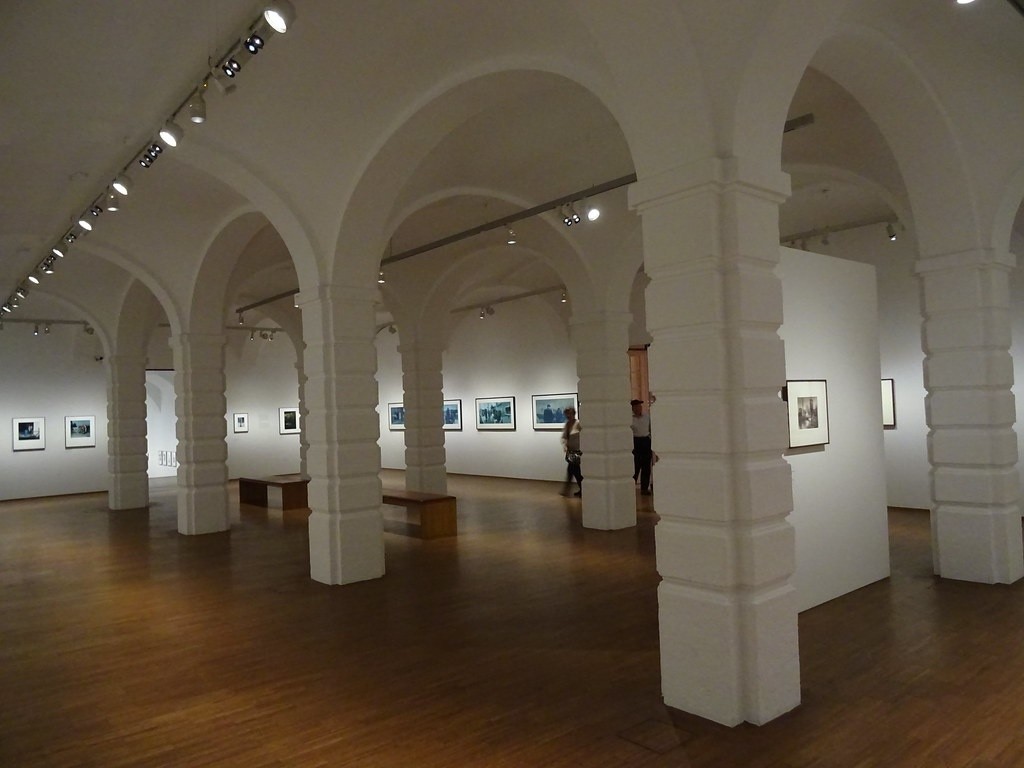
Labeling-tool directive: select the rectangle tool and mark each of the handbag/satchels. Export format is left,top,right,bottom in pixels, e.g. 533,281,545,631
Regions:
651,450,659,467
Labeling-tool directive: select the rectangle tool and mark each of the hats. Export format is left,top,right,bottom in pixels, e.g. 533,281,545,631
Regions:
631,399,644,405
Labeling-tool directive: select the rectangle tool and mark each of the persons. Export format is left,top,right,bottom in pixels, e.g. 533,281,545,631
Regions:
630,399,653,496
543,405,563,423
557,408,583,497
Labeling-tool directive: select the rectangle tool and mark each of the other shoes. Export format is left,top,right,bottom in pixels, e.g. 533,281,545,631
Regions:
640,490,651,496
559,489,572,497
574,490,582,497
566,447,582,465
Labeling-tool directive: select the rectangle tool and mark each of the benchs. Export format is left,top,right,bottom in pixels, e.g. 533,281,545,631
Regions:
239,476,311,510
379,487,456,540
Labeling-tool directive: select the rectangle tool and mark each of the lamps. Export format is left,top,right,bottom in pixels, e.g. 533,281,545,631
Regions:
377,269,385,285
481,305,495,319
507,224,517,246
236,293,297,343
561,291,569,304
190,0,298,125
552,195,601,227
136,122,183,170
388,323,397,333
0,176,131,336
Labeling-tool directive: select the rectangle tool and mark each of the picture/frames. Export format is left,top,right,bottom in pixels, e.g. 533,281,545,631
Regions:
787,380,830,448
475,397,516,430
531,393,578,430
278,406,301,434
13,417,46,451
441,399,463,431
882,378,896,425
64,416,95,447
388,403,407,430
233,412,249,433
